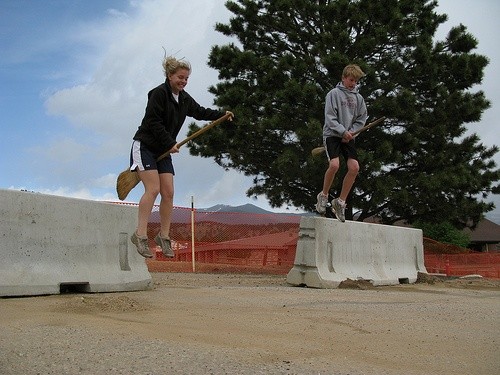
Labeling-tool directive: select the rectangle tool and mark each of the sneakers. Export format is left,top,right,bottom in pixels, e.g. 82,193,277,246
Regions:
316,191,328,214
130,230,153,258
331,197,346,223
154,232,175,258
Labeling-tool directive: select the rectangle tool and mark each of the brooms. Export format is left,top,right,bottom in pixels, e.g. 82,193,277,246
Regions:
312,116,385,160
116,112,233,200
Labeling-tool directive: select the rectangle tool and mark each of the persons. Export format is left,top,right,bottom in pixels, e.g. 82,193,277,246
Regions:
129,57,234,257
316,64,367,222
